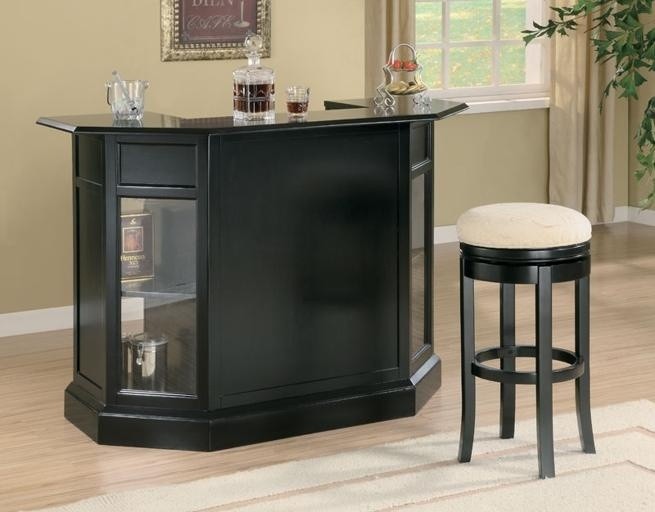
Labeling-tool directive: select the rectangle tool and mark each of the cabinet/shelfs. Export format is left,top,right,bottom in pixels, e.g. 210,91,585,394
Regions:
31,92,468,450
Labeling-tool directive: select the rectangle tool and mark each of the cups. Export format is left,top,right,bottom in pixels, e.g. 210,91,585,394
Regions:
286,86,309,117
105,79,147,120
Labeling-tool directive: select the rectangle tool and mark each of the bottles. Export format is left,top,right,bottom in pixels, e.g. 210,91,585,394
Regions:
233,34,274,118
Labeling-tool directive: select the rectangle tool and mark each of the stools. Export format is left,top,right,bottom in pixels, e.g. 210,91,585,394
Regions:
454,201,598,481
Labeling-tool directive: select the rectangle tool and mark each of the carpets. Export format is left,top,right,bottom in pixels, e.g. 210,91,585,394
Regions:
39,395,655,512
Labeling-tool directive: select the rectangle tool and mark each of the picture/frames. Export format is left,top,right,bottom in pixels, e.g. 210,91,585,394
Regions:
156,1,275,65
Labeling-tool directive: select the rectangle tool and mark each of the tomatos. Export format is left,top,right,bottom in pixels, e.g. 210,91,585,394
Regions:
389,60,417,69
